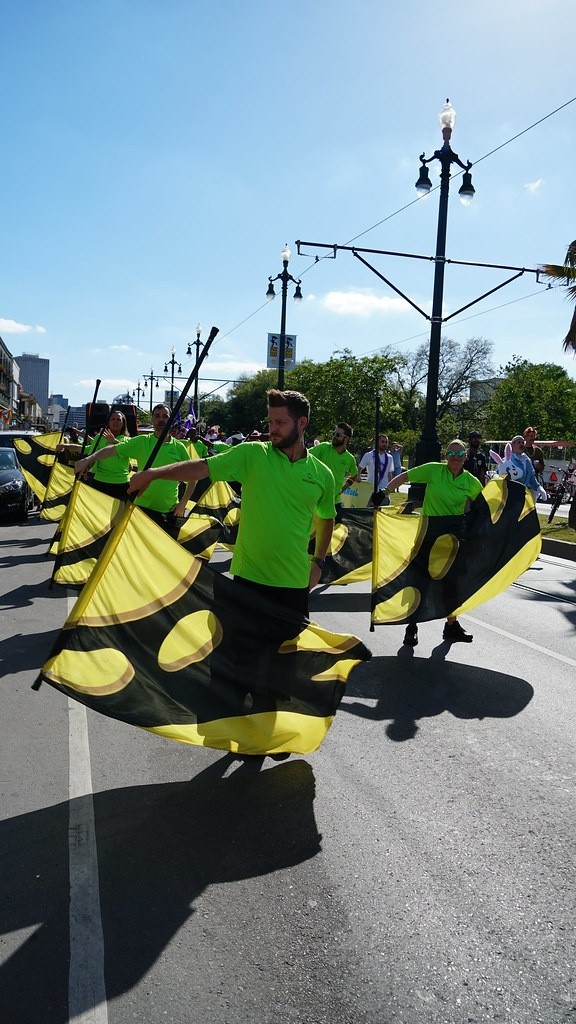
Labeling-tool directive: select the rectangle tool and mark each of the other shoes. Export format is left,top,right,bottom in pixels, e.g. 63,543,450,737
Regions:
403,625,418,646
442,621,473,642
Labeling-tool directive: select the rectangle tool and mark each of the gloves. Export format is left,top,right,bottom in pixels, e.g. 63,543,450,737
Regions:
371,487,390,507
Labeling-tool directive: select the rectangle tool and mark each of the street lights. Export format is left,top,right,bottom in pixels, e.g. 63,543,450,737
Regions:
131,379,146,408
413,399,420,433
143,366,161,414
460,392,468,441
436,396,442,421
184,322,209,434
163,345,183,409
414,93,475,466
265,242,303,391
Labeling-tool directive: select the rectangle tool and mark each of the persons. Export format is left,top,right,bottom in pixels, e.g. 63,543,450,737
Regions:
357,433,394,492
169,417,269,457
56,410,132,502
462,431,489,487
63,422,93,445
366,440,484,645
524,427,545,483
74,404,192,532
387,441,404,493
307,422,358,580
490,435,547,560
127,388,337,764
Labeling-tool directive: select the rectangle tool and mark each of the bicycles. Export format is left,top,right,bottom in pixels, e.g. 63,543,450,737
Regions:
546,468,576,524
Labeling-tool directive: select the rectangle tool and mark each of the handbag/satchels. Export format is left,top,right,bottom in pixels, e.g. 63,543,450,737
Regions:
536,486,548,502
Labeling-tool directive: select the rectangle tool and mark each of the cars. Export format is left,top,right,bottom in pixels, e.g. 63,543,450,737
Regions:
0,446,36,524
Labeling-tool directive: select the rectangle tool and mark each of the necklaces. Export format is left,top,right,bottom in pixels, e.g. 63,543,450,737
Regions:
526,445,535,458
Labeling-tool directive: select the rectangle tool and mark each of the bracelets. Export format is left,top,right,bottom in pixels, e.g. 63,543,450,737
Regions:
347,479,354,486
311,556,325,569
384,486,390,493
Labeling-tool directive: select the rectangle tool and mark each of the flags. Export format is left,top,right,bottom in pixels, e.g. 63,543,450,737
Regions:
369,474,543,626
39,451,89,523
41,503,372,755
186,472,241,551
183,403,196,428
318,503,410,584
51,479,224,590
186,443,200,460
14,429,65,505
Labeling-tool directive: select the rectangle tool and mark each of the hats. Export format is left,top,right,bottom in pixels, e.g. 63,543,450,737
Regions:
231,433,246,440
512,436,525,443
469,431,482,440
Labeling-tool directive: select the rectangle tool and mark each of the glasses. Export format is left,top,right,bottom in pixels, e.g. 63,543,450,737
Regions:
446,450,465,459
333,431,350,439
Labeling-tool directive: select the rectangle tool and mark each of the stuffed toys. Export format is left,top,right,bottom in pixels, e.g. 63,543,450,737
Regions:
488,442,523,481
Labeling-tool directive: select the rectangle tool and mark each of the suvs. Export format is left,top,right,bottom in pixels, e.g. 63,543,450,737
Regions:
0,430,44,454
